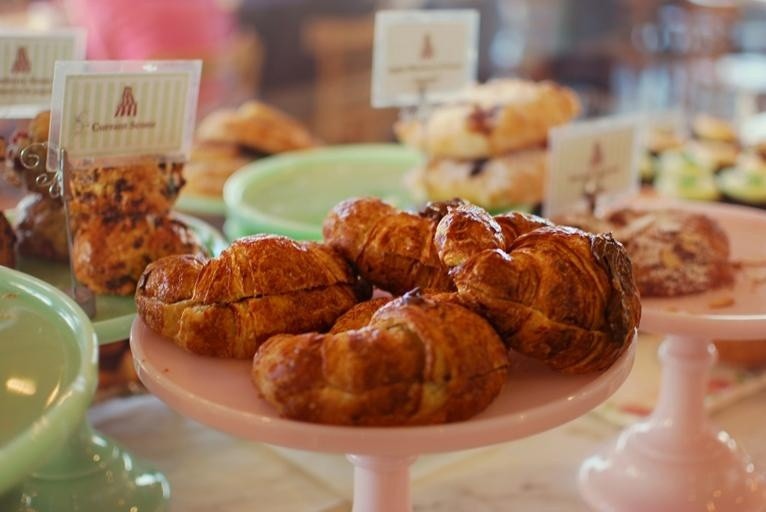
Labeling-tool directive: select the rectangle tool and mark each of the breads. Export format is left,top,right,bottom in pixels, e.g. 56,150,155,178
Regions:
11,103,731,425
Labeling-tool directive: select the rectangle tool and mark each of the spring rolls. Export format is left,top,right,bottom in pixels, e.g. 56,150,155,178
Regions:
406,79,580,155
